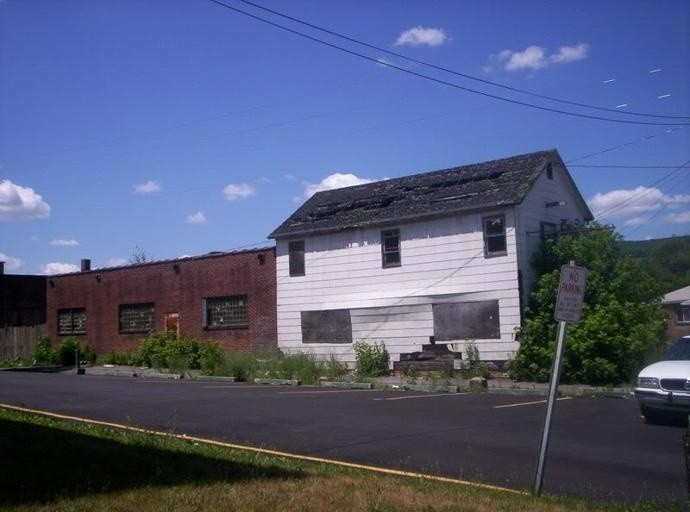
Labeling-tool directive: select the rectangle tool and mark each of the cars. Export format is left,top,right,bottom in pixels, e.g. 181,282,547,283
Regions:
634,334,689,423
